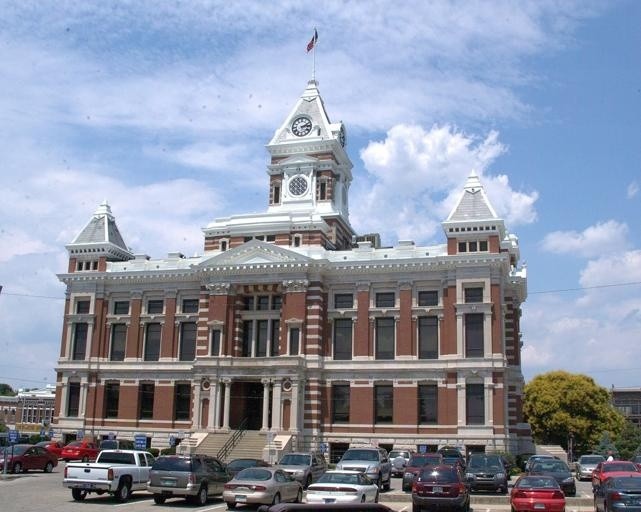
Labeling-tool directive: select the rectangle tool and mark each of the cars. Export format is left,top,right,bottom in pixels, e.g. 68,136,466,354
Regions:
510,454,576,512
574,456,641,511
0,440,101,474
227,458,396,512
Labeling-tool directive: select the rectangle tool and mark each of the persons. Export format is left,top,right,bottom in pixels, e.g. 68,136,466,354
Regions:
607,449,614,463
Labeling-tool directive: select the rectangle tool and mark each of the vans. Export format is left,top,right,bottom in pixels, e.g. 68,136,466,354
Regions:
100,439,134,450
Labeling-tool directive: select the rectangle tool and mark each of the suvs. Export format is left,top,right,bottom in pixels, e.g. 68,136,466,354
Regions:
336,447,511,511
148,453,233,506
275,454,329,488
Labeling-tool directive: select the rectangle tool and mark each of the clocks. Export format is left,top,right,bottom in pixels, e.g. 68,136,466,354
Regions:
291,117,312,136
340,127,346,147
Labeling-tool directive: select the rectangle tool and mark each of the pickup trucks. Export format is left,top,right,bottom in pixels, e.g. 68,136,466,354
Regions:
63,448,156,503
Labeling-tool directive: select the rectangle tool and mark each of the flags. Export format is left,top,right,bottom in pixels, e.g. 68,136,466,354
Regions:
307,30,318,52
